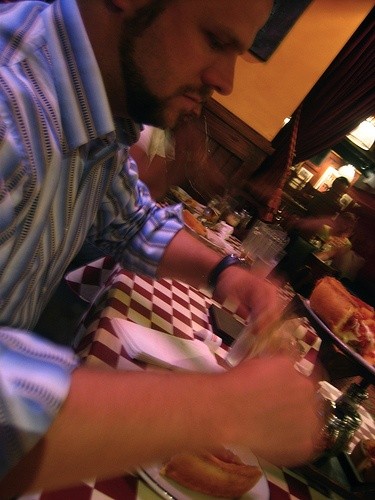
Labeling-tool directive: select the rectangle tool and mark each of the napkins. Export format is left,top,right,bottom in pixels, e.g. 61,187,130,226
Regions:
111,317,218,374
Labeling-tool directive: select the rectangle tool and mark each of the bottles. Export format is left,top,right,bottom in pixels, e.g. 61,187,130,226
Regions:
204,193,252,236
317,382,369,453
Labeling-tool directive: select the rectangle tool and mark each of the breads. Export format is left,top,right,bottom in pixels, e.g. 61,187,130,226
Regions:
161,446,262,499
308,275,375,369
182,210,207,237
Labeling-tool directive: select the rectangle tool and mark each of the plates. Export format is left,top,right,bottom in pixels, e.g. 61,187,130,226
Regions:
136,442,271,500
170,190,201,215
184,222,224,250
298,293,375,376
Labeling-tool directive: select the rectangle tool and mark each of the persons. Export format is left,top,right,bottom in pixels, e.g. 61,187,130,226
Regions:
0,0,325,489
286,176,357,262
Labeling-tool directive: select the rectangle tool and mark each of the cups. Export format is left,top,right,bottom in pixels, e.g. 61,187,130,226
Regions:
226,325,259,368
237,219,288,279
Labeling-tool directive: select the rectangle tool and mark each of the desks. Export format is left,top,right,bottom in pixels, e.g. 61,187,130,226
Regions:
50,200,375,500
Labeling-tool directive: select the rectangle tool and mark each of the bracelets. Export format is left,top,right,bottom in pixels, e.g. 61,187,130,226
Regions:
207,252,252,289
